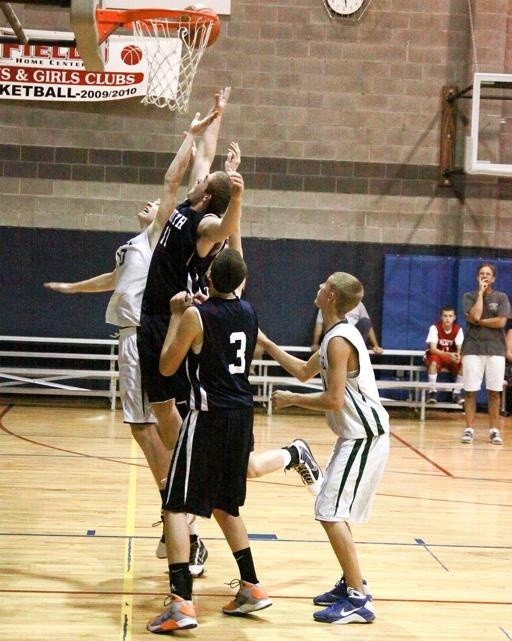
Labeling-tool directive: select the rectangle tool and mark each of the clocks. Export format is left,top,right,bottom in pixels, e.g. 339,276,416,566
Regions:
321,0,376,25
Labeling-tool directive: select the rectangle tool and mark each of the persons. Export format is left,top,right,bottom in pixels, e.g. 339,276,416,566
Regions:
423,307,465,404
312,301,383,355
501,322,512,416
45,85,324,633
461,264,512,445
257,271,390,624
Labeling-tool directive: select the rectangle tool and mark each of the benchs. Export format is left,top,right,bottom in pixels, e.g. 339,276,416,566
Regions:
1,330,509,424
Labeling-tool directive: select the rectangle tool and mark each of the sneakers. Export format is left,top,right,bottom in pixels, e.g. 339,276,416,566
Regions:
488,427,504,445
461,427,474,444
147,593,198,633
426,392,440,407
290,438,324,494
188,534,209,577
222,579,273,614
313,578,376,625
156,514,199,559
455,394,465,404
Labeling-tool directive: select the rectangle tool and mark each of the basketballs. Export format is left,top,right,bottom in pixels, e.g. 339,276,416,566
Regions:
181,5,220,48
